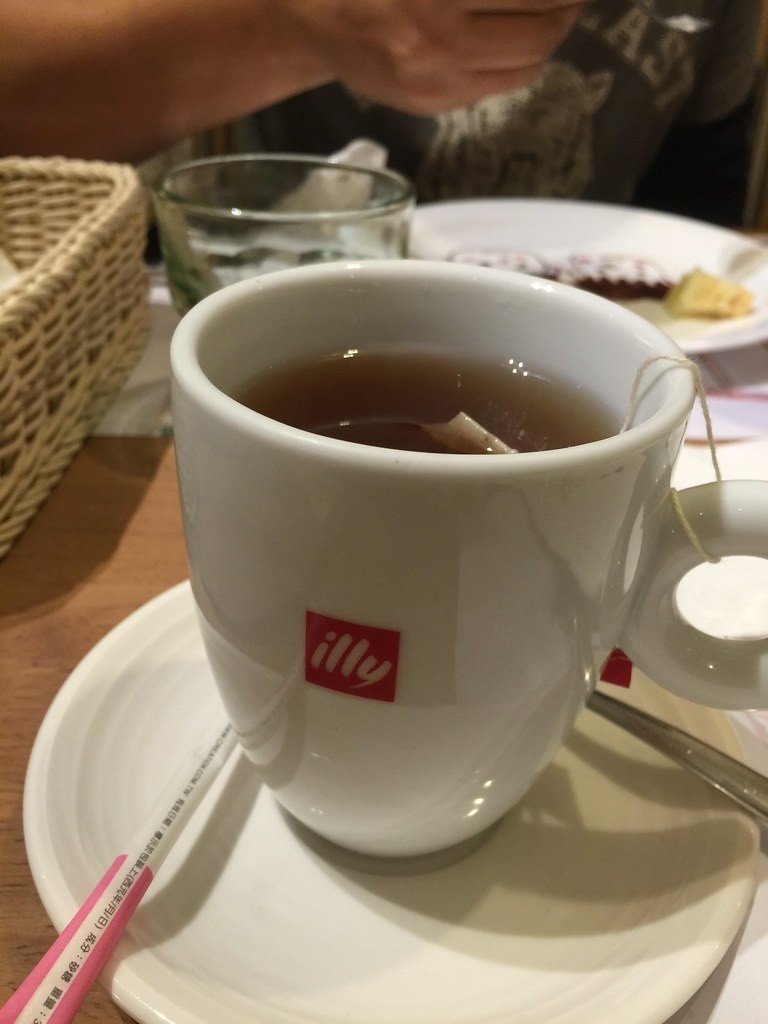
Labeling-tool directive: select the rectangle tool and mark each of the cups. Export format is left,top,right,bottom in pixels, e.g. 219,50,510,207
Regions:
150,153,416,344
163,257,697,857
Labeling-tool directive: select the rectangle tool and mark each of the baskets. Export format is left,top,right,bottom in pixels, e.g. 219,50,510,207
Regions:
0,157,152,559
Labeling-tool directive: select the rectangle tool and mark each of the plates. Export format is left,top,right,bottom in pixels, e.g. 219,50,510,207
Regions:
397,197,768,365
19,576,766,1023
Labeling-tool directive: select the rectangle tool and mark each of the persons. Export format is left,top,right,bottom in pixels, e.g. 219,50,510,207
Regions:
0,0,768,246
